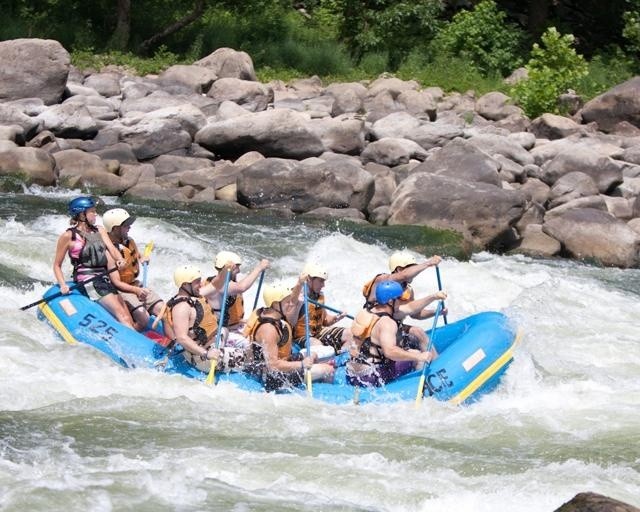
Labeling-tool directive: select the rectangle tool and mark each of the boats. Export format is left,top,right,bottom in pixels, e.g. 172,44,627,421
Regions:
37,281,524,413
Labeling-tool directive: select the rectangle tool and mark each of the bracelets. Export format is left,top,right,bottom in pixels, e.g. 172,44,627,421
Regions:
201,350,208,361
302,361,304,368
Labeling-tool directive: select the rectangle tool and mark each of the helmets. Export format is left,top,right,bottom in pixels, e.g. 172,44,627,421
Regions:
389,250,419,273
174,264,202,288
299,263,328,284
69,197,96,214
263,282,293,308
214,250,244,270
102,207,132,232
375,278,403,304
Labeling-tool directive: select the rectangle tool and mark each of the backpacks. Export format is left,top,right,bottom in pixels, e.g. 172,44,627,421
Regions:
72,226,107,267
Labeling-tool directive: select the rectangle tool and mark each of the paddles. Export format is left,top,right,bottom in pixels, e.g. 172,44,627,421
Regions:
415,300,443,404
141,242,154,288
204,269,231,384
303,281,314,396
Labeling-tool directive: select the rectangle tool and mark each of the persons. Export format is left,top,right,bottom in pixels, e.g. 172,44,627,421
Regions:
202,251,271,348
346,280,448,388
362,250,448,361
53,197,135,327
285,262,352,355
166,265,245,373
244,272,335,384
103,207,175,341
